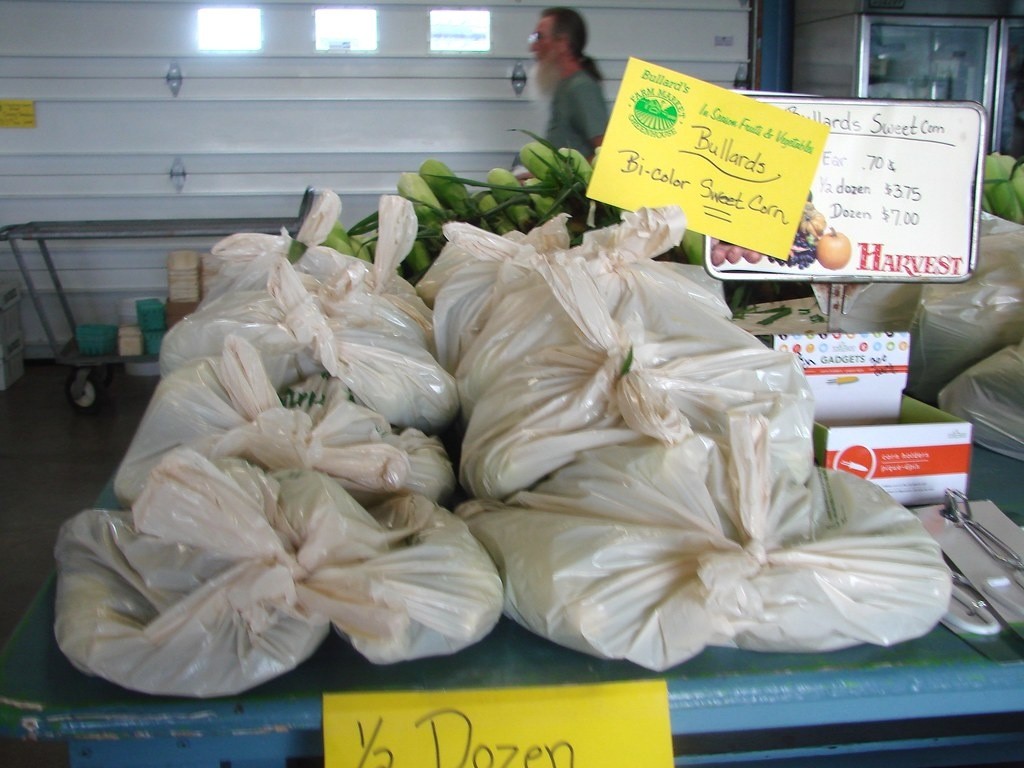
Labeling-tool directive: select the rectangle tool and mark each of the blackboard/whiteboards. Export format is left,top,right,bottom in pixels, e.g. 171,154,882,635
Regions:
703,89,986,285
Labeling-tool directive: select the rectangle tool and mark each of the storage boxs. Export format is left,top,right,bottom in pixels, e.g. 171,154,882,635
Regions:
74,251,215,357
768,330,971,505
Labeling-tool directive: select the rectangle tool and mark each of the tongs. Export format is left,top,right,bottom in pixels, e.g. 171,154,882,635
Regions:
944,487,1024,584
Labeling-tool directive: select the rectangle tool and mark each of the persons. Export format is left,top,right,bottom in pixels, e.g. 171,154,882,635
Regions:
528,7,610,162
